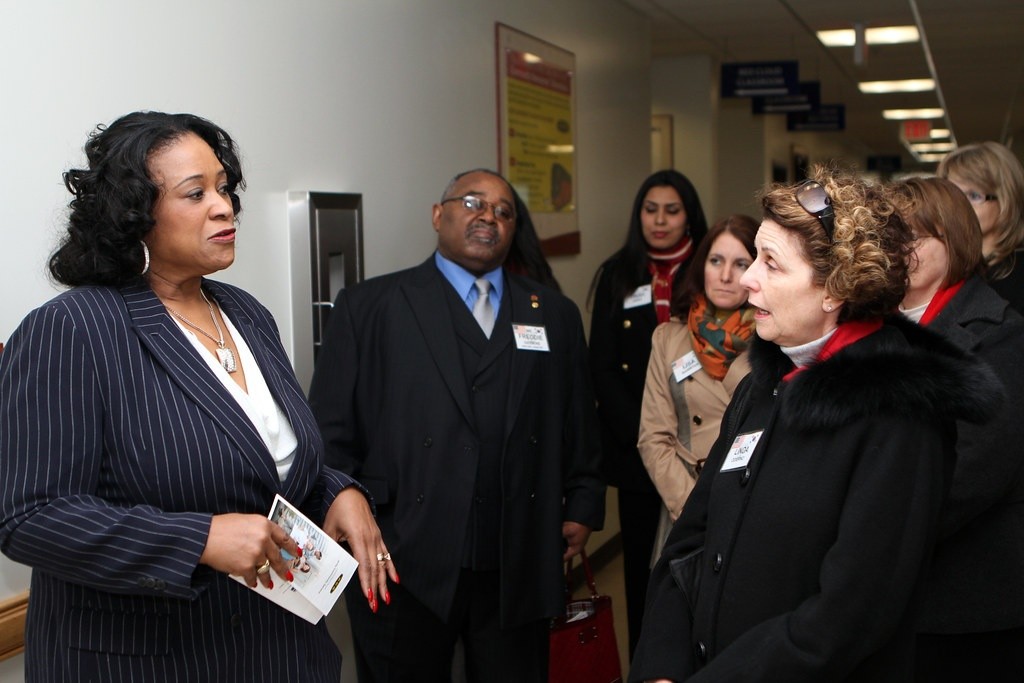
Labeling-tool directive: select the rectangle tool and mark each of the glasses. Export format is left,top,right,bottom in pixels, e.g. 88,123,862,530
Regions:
440,195,518,224
962,190,998,207
911,232,944,248
793,176,834,248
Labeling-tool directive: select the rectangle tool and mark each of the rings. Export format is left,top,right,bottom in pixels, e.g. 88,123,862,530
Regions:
377,553,386,561
385,552,392,561
257,559,270,574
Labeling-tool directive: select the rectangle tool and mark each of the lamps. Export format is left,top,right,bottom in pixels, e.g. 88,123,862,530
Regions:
842,23,888,68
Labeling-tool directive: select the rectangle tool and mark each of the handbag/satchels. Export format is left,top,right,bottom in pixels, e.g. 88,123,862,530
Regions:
549,548,625,683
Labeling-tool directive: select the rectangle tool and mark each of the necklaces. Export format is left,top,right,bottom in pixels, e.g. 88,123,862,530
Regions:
160,280,236,375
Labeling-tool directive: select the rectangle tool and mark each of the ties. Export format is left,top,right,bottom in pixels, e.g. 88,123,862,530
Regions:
473,278,497,341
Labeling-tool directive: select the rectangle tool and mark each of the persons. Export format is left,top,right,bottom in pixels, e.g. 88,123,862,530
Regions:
884,170,1024,446
939,142,1024,319
636,210,782,609
581,168,712,670
304,167,592,683
1,111,399,681
273,511,324,574
622,168,1024,683
